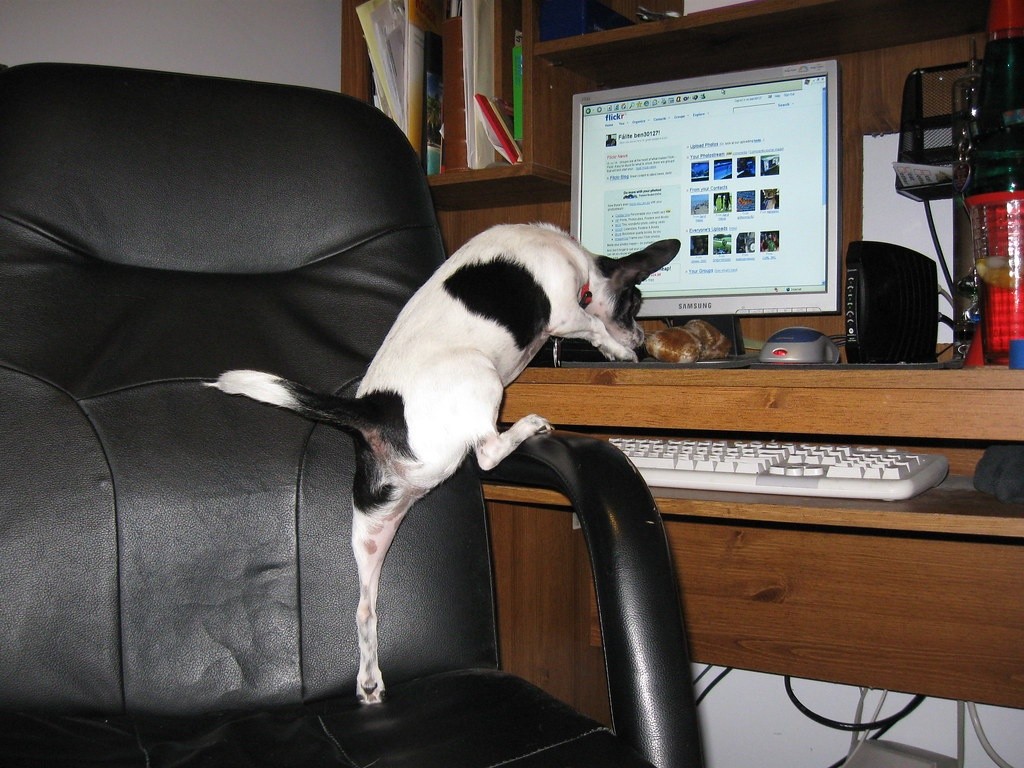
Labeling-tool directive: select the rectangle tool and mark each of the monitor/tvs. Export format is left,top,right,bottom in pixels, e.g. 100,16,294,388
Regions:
571,59,845,368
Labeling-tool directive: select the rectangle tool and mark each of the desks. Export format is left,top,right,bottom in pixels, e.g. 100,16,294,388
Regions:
482,368,1024,731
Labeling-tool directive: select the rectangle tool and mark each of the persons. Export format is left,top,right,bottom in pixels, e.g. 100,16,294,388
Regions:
761,189,779,209
720,239,730,254
762,233,779,252
691,238,707,255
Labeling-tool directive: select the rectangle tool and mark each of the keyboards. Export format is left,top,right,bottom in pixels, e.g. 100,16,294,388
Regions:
609,436,949,502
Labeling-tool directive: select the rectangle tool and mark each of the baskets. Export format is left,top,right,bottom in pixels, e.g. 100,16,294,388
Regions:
895,61,983,200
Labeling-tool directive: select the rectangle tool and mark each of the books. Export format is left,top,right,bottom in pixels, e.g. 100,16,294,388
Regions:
356,0,523,176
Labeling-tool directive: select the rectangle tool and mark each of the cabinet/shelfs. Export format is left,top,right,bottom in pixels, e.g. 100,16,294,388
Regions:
341,0,533,177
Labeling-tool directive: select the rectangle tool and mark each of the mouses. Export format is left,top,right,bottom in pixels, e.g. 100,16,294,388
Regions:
758,327,840,364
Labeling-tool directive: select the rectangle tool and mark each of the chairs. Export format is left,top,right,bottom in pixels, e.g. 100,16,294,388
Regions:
0,62,703,768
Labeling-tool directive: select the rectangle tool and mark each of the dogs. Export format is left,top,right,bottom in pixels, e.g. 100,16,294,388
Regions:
202,220,682,709
